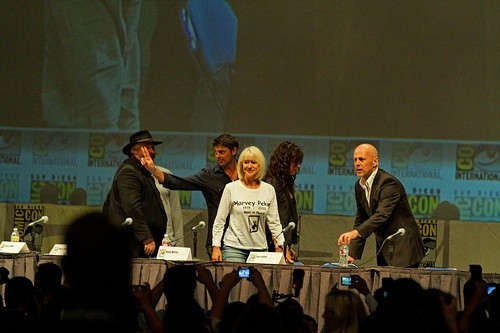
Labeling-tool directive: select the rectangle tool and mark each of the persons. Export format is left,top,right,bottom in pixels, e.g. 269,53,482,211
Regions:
41,0,140,135
102,129,185,258
0,213,500,333
141,134,240,260
263,140,304,264
337,143,425,268
211,146,295,263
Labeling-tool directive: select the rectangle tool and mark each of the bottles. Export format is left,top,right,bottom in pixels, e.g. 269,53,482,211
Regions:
162,234,170,247
10,228,19,242
340,242,349,268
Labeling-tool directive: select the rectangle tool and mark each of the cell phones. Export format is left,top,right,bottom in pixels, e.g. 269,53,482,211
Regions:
341,276,354,286
238,268,250,278
487,285,496,295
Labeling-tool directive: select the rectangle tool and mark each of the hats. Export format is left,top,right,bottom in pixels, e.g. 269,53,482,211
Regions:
122,129,162,157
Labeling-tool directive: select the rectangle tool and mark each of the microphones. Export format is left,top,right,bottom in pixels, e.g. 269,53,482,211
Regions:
281,222,296,233
121,217,133,226
27,216,49,228
192,220,206,230
386,228,406,240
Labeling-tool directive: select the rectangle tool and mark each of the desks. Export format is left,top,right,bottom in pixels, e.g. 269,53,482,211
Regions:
0,252,64,306
131,258,473,333
